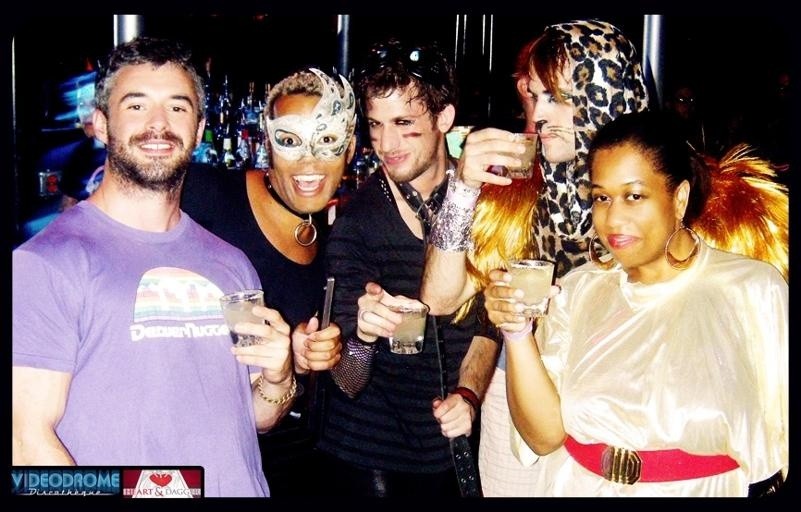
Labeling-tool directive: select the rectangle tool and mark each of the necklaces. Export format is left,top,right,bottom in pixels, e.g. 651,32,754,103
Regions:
259,174,330,250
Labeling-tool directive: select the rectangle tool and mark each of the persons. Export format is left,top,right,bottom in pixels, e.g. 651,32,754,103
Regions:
315,35,506,499
11,35,299,498
57,75,109,213
181,59,361,495
667,75,702,151
474,112,790,497
418,19,788,497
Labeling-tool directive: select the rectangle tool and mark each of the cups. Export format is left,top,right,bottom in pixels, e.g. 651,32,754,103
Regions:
508,258,555,317
219,288,265,349
387,302,429,355
505,132,538,180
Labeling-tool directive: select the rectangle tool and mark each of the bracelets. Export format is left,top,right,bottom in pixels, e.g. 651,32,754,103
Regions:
262,366,292,385
428,172,481,253
259,374,297,406
493,317,534,341
450,386,483,413
